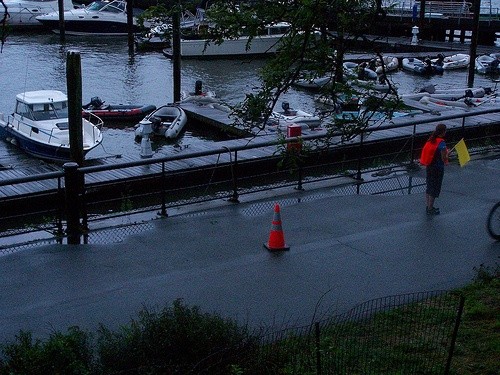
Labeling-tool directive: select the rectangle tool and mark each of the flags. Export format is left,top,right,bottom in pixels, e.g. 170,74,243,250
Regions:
455,140,470,166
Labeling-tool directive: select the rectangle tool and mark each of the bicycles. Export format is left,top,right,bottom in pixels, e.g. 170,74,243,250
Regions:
486,202,500,241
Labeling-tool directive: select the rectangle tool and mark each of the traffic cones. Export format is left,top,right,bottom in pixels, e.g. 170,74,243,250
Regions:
262,204,290,252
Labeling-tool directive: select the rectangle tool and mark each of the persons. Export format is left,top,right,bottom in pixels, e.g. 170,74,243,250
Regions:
421,123,449,216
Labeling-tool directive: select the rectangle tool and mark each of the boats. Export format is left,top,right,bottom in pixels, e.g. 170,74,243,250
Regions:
132,102,188,143
416,86,485,100
35,0,148,36
419,95,489,110
150,8,221,37
342,61,398,92
427,53,470,70
475,53,500,75
160,17,329,58
281,75,331,90
0,0,76,27
401,57,429,75
261,101,323,132
333,109,409,123
0,89,104,164
362,55,398,74
82,96,157,120
313,92,360,114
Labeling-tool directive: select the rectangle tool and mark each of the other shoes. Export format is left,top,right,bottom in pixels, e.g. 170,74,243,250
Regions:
426,206,440,216
